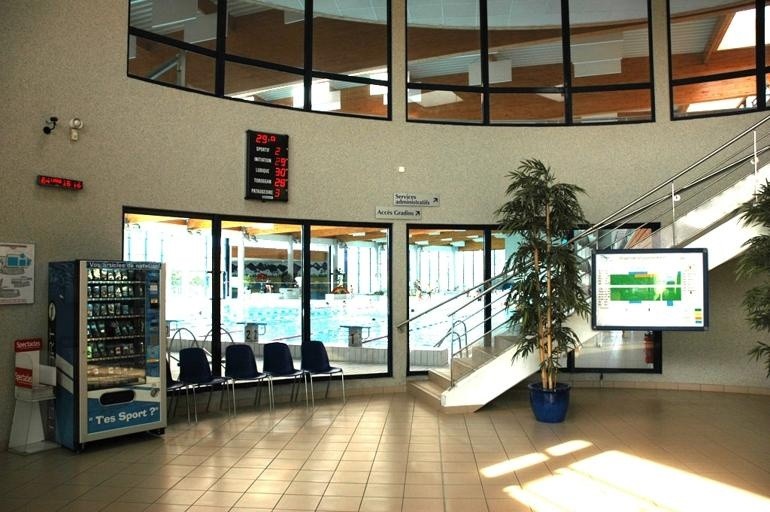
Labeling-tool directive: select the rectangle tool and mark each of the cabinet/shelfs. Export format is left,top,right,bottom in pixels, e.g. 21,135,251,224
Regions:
47,259,168,454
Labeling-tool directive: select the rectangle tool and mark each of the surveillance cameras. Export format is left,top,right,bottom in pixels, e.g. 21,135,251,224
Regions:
43,115,59,134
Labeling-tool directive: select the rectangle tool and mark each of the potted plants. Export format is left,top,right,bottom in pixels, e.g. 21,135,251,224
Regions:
492,158,595,425
732,179,770,378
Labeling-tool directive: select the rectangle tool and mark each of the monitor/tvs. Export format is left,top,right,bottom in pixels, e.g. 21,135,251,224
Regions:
591,247,709,332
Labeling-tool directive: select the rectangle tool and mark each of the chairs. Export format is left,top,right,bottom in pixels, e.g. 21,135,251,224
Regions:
179,347,232,424
300,341,346,409
226,344,272,416
262,341,310,412
164,359,191,425
